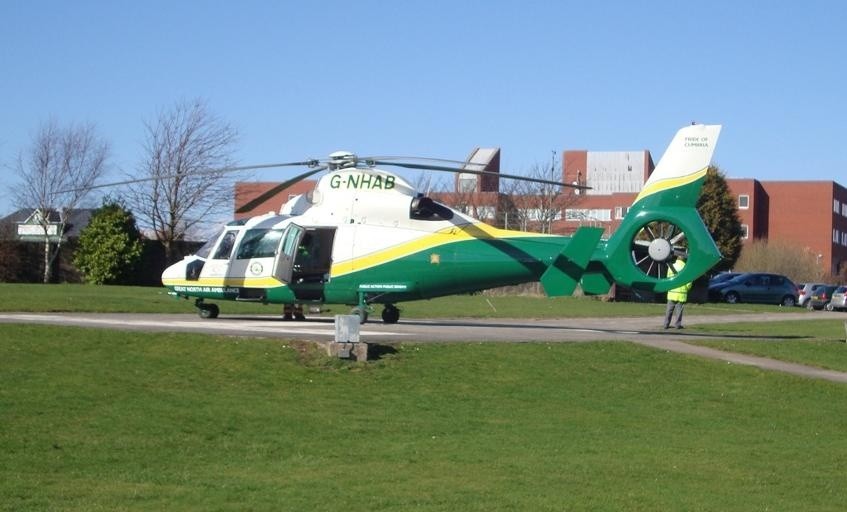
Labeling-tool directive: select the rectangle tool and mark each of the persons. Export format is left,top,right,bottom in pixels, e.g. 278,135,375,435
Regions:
660,254,693,330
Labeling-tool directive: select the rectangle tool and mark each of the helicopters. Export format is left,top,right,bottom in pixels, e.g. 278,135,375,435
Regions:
49,121,725,325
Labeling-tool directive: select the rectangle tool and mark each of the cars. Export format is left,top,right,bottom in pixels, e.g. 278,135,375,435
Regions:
688,271,847,318
